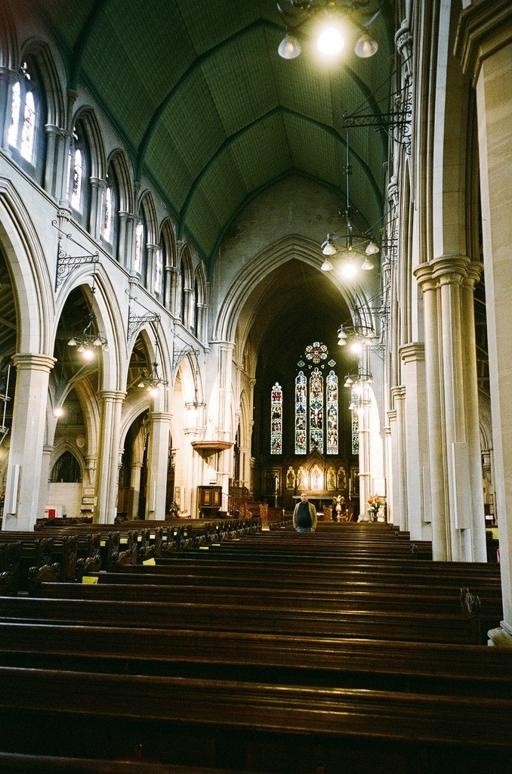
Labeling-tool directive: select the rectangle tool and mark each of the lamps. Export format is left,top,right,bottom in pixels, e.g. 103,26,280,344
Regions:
343,356,375,388
185,354,207,410
320,127,381,271
349,393,373,411
337,311,379,347
67,262,111,352
137,323,171,391
274,1,382,59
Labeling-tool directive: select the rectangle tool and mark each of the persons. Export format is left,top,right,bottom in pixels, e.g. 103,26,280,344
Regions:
292,493,317,532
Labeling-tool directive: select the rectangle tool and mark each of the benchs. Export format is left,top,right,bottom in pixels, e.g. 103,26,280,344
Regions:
0,517,512,774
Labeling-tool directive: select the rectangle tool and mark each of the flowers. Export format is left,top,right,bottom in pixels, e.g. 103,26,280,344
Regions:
368,496,386,514
